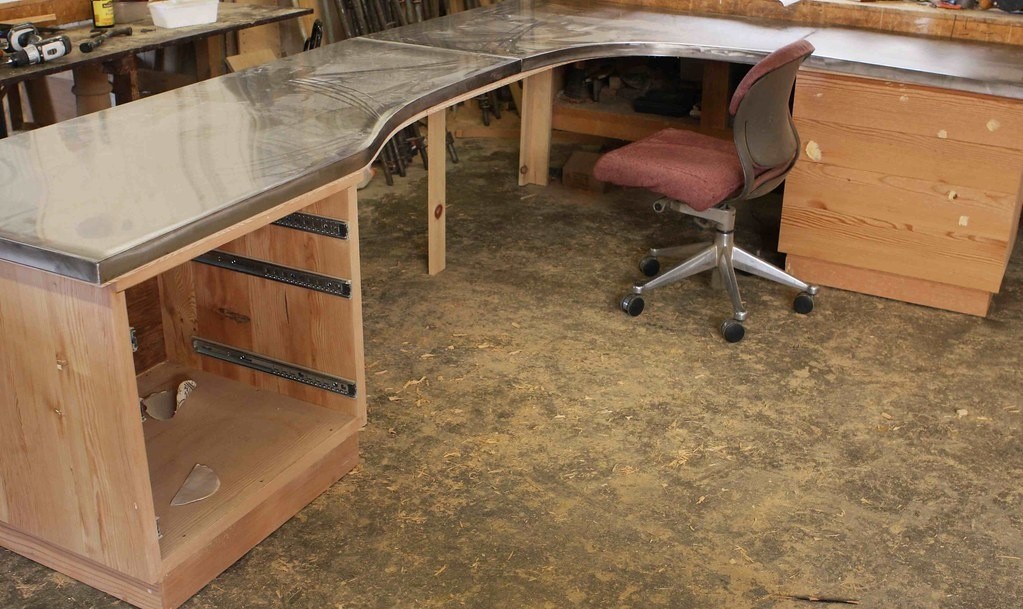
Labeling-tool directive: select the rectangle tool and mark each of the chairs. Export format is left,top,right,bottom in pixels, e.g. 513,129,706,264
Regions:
593,38,821,342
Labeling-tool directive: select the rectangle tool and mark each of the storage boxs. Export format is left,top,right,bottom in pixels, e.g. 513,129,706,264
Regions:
146,0,219,29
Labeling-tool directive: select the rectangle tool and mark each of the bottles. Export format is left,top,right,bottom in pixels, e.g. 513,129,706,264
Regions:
90,0,116,28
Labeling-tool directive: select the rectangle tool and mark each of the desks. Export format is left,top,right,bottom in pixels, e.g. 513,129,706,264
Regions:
0,37,523,609
0,0,314,138
355,0,1023,317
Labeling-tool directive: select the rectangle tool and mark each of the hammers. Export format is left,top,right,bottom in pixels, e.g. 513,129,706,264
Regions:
78,26,133,53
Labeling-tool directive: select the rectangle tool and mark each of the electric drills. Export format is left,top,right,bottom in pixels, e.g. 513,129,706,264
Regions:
0,22,73,68
